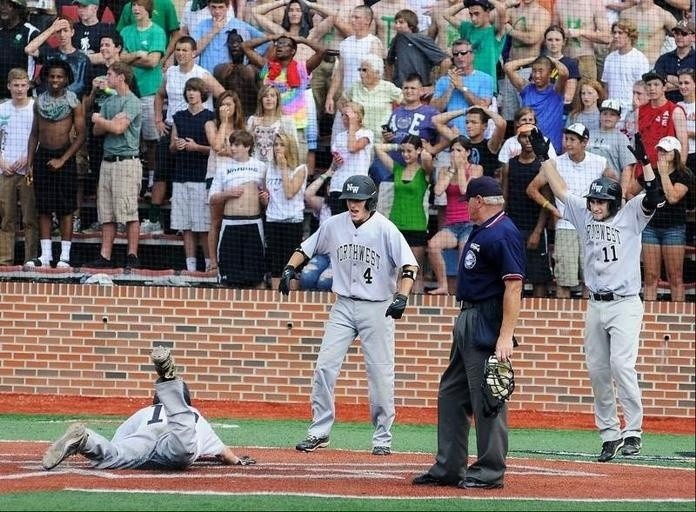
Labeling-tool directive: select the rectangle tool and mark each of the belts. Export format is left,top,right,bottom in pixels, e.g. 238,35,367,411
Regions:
103,155,139,162
589,293,614,301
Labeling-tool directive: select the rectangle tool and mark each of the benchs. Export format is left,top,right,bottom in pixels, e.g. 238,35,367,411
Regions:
0,198,696,295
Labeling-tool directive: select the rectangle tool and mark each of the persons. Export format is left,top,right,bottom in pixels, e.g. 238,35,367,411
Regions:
407,173,530,492
275,174,422,458
526,120,669,465
37,344,258,473
1,1,696,304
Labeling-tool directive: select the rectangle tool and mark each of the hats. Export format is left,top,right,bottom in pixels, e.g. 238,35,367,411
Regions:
642,69,667,85
672,20,696,33
463,0,490,8
600,99,621,115
562,123,589,140
458,176,503,202
72,0,99,6
655,136,681,152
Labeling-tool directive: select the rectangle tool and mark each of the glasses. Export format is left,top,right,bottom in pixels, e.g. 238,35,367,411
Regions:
453,51,472,56
358,68,366,71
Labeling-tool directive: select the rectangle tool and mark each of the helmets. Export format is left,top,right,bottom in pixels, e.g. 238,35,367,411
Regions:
338,175,377,211
583,177,621,209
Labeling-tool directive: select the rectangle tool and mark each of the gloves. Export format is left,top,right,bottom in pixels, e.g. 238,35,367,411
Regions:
385,294,407,319
528,128,550,162
278,265,299,296
627,132,649,166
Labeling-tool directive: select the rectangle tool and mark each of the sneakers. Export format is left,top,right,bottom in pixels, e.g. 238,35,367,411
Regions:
412,474,504,490
597,437,642,462
81,254,139,268
42,423,88,469
296,434,329,452
373,446,390,455
23,258,71,268
151,346,174,379
